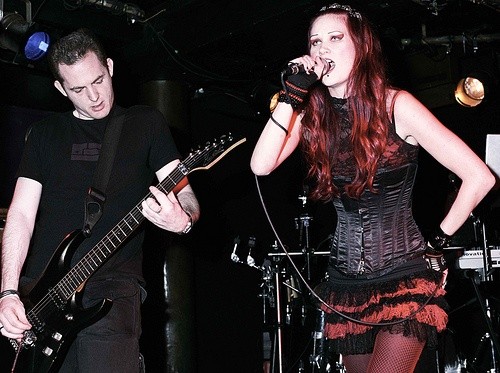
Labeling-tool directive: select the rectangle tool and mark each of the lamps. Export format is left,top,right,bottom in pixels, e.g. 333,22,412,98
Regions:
448,64,500,113
0,11,51,69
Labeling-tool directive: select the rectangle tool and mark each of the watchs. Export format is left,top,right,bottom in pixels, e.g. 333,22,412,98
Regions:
183,213,192,233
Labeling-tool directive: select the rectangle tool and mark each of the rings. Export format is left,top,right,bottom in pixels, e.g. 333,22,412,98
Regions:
0,326,4,330
156,207,162,214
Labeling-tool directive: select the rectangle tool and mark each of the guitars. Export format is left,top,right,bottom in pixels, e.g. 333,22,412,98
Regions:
0,128,246,373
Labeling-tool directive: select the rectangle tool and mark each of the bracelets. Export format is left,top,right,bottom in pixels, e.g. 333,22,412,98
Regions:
0,290,19,299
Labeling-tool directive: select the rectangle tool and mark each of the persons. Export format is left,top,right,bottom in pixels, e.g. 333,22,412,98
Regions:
250,3,495,372
0,32,200,373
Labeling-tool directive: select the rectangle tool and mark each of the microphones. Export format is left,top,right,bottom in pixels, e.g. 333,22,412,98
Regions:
289,58,328,75
